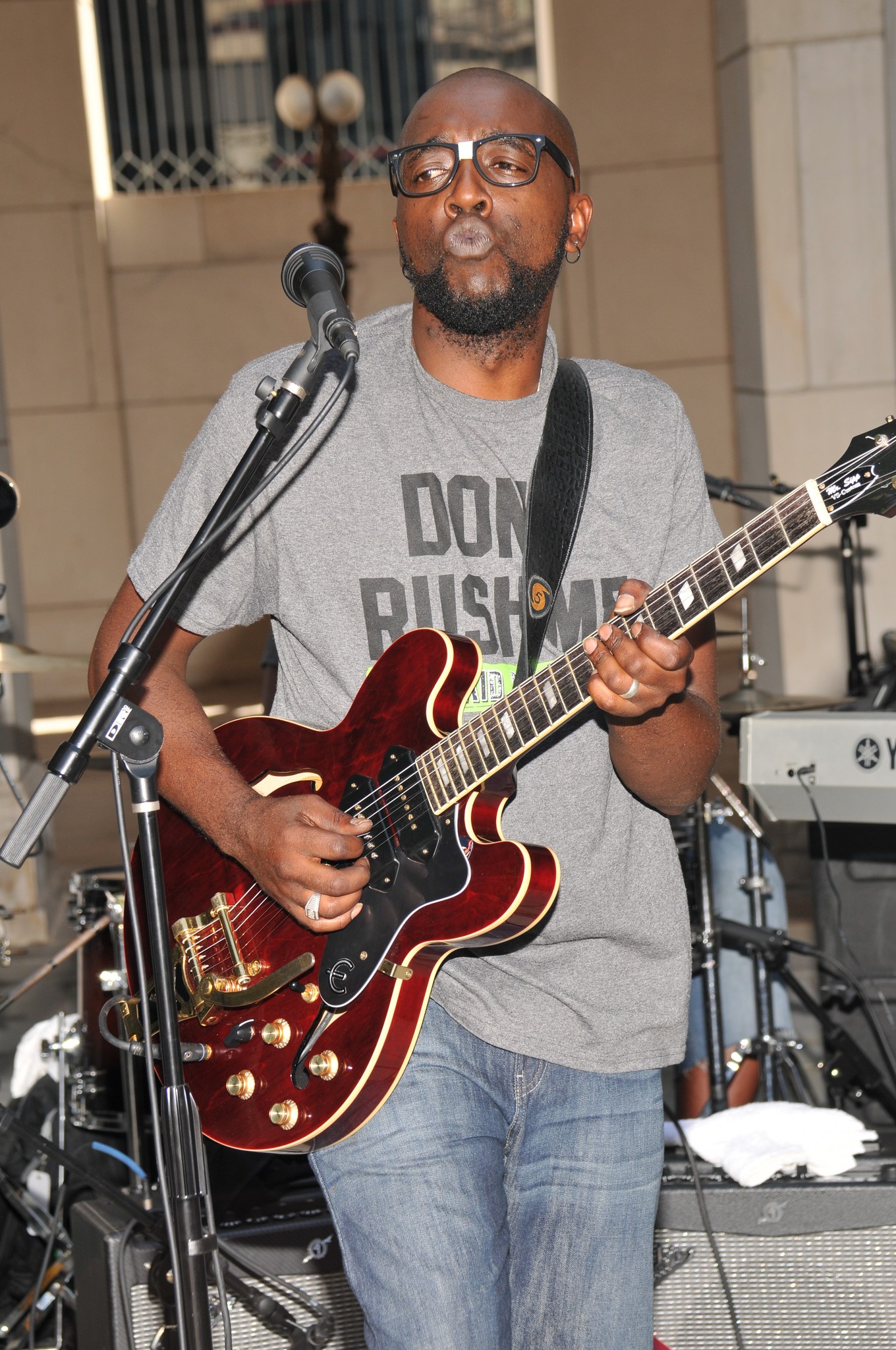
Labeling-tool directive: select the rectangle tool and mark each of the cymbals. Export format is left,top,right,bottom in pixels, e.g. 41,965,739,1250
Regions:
713,685,861,724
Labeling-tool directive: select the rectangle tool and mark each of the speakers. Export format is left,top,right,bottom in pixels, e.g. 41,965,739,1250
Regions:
809,817,896,1146
650,1177,896,1350
72,1176,368,1350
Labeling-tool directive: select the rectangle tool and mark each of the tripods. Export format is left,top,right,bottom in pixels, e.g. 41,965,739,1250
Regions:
699,788,819,1122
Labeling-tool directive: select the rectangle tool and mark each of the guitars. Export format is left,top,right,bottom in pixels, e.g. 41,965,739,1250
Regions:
120,415,896,1155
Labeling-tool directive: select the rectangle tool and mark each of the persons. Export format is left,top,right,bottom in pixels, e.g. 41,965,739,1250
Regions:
672,805,791,1116
89,68,723,1349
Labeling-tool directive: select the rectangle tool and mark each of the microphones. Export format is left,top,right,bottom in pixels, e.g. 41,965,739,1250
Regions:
290,1316,335,1350
705,477,765,511
713,919,811,955
281,242,359,363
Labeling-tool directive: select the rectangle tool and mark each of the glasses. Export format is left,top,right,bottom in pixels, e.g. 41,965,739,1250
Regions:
386,128,578,198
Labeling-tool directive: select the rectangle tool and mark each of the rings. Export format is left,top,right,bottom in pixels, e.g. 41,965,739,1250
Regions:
305,892,321,920
619,679,640,699
